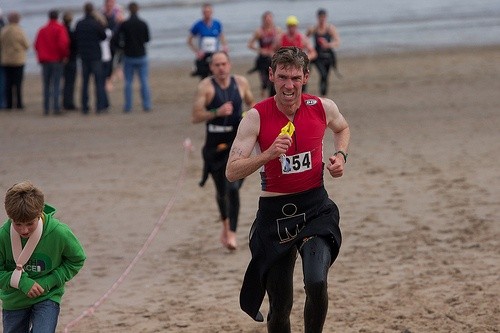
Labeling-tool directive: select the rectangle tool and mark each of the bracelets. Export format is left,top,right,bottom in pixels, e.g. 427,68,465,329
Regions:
333,150,349,163
214,108,217,117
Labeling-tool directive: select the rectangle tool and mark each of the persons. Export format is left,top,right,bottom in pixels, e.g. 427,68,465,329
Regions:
0,180,87,333
0,10,30,111
225,47,351,333
72,2,112,114
98,0,125,91
304,8,340,98
60,10,79,110
247,10,281,100
186,2,228,80
271,15,317,93
115,1,153,112
190,50,255,255
34,8,70,116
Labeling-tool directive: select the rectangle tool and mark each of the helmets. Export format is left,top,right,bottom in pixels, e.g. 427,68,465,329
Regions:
285,15,298,26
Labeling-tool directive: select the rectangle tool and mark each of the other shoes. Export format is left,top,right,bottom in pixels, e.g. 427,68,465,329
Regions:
220,231,238,251
